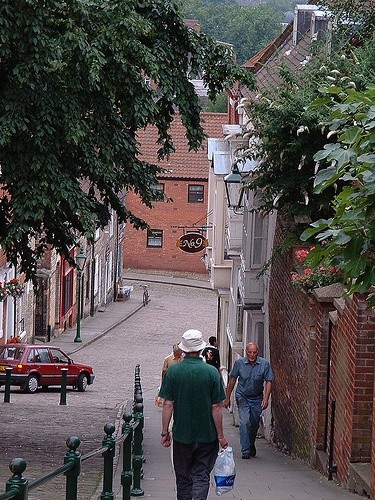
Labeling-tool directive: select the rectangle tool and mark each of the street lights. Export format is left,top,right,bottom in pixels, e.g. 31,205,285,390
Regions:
73,246,87,343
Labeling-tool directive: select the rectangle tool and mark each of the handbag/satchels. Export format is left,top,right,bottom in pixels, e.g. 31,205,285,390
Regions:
214,447,236,496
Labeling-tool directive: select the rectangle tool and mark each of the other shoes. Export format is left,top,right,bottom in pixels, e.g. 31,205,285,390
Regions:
241,444,256,459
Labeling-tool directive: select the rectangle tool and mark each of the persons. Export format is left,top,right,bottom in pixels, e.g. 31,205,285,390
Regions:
200,336,221,375
158,329,229,500
223,342,275,459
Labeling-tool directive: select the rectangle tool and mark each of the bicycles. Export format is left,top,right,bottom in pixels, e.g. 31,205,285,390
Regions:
139,285,150,307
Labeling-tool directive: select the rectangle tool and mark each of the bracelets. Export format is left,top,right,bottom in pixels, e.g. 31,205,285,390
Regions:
218,436,224,439
160,431,170,437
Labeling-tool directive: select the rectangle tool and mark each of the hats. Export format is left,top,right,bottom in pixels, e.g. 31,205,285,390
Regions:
178,329,206,352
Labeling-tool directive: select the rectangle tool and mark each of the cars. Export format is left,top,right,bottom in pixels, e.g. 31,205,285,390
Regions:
0,343,95,394
